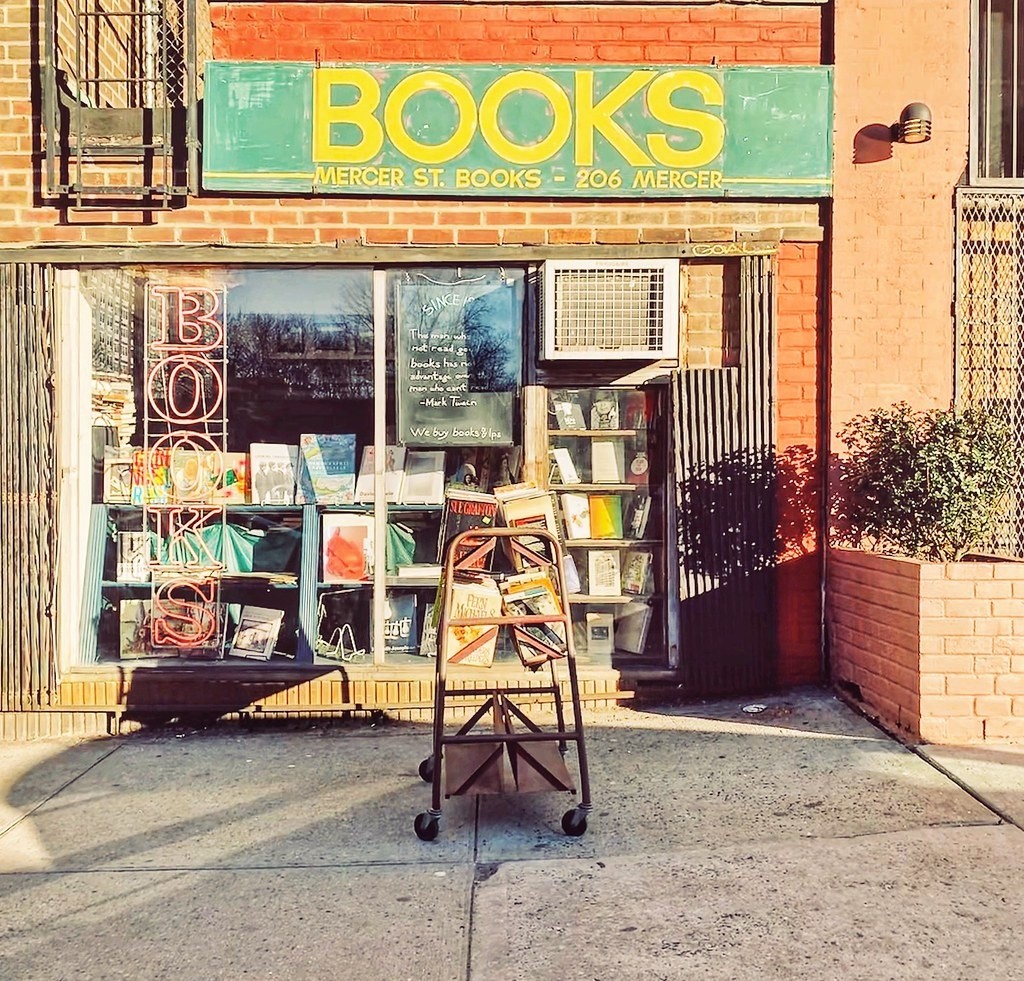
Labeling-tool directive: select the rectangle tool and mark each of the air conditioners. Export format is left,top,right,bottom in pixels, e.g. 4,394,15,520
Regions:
534,257,680,362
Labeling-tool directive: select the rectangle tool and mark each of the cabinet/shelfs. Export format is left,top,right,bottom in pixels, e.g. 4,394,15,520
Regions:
96,386,677,675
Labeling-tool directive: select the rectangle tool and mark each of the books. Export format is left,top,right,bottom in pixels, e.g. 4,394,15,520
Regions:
101,387,666,675
88,368,137,448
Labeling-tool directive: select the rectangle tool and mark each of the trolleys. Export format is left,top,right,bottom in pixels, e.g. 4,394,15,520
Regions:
414,525,593,840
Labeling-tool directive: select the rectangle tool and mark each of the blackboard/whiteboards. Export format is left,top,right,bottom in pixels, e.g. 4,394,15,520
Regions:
393,268,514,446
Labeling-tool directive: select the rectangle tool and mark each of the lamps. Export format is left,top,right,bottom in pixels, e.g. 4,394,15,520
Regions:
891,101,932,145
